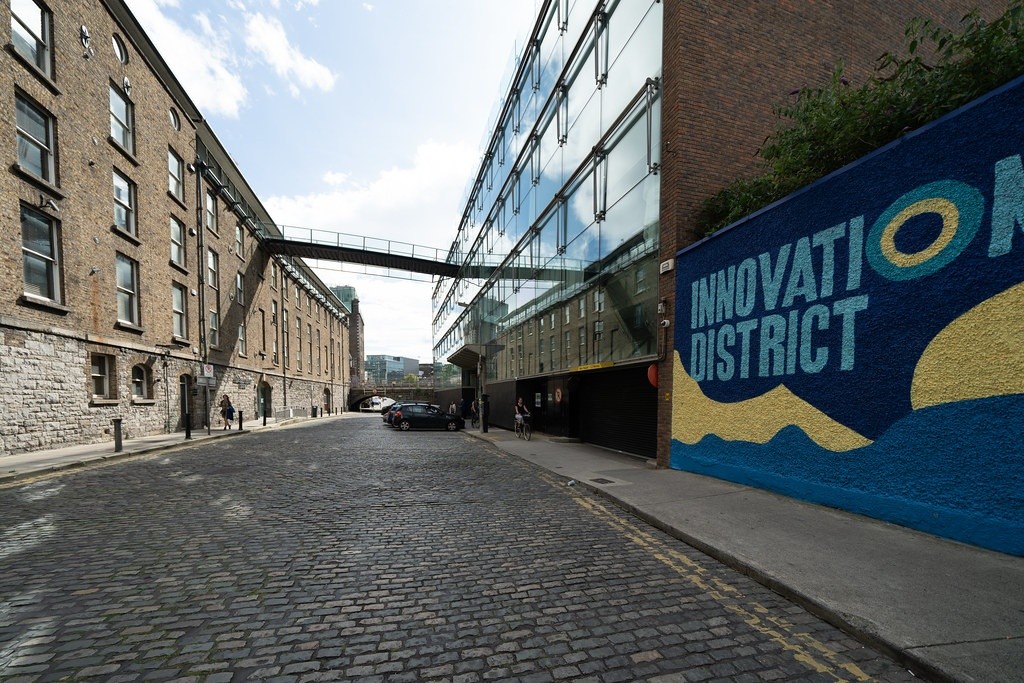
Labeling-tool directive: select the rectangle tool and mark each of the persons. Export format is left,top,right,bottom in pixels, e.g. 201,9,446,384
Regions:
458,399,468,419
514,397,532,435
471,398,479,425
448,401,456,414
371,401,375,409
217,394,235,431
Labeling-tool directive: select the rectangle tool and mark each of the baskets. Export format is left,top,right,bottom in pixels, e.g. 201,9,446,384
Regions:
523,415,533,423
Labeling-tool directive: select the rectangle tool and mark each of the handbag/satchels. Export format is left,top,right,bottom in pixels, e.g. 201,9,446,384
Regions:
220,410,224,414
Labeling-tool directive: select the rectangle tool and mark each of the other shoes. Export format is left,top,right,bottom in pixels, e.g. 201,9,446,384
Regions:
229,424,231,429
224,426,226,430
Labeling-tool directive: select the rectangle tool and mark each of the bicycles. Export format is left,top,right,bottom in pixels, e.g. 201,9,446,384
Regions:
471,408,480,428
514,414,531,441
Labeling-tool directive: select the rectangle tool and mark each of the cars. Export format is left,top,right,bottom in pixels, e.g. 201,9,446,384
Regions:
392,404,465,431
381,401,441,428
